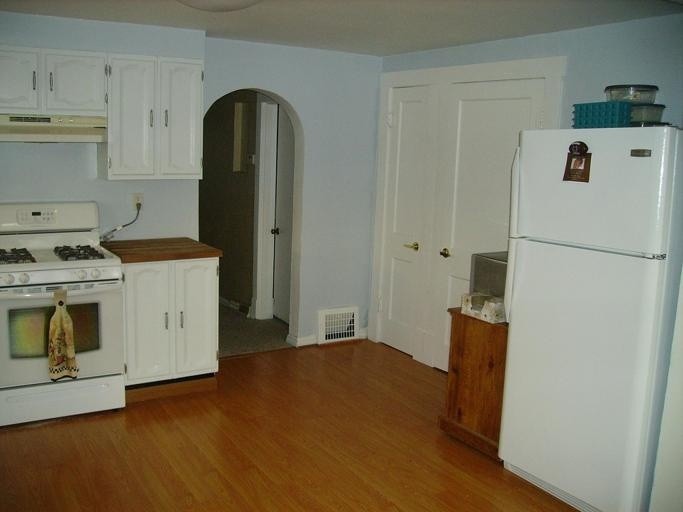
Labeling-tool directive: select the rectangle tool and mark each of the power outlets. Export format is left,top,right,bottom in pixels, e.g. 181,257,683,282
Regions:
130,192,144,211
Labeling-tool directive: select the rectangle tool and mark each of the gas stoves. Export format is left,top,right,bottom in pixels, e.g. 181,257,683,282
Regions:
0,233,123,285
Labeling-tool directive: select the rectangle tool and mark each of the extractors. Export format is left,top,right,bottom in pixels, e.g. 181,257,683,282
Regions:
0,115,108,143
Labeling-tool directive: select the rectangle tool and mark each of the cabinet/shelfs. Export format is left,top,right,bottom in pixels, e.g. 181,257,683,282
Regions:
96,54,203,181
99,237,223,390
0,46,107,117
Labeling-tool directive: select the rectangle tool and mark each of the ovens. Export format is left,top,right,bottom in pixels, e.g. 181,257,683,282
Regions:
1,281,125,426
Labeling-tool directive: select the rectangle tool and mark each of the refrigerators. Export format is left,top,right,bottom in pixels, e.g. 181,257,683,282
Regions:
497,125,683,509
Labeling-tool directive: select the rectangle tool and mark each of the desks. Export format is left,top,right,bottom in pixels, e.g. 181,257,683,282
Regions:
438,306,508,465
